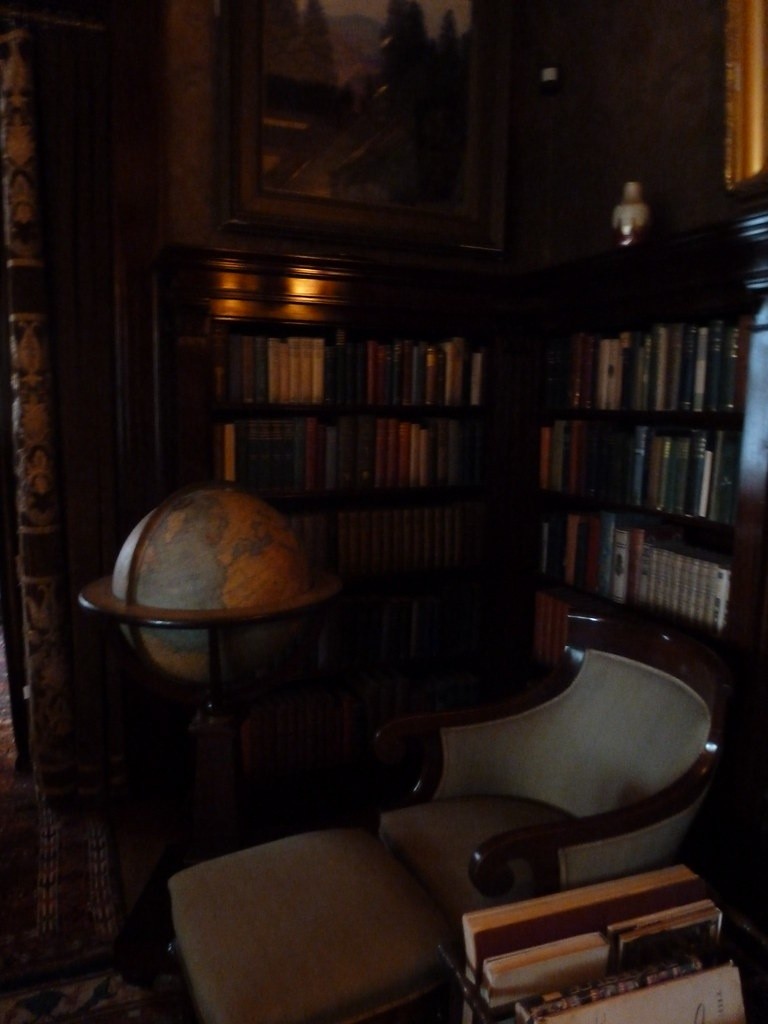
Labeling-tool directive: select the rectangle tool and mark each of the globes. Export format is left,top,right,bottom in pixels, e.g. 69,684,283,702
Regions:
78,482,342,997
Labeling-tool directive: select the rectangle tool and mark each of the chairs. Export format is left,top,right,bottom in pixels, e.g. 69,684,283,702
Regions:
373,605,732,919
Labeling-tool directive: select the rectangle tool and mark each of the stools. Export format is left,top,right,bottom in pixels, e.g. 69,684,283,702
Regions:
167,829,459,1023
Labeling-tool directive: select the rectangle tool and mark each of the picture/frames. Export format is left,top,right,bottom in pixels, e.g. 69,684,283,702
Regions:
220,0,510,252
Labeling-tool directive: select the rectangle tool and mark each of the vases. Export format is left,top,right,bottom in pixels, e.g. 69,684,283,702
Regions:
613,182,655,247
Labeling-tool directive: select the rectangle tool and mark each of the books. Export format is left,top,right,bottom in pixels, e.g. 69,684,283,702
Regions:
212,311,741,790
459,861,748,1024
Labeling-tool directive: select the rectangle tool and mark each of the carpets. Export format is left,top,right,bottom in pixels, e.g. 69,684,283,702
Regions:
0,723,126,988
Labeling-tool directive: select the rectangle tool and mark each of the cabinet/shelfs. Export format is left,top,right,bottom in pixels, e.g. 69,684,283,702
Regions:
146,244,768,824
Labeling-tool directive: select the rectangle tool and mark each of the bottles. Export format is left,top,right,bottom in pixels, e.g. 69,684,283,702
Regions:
612,181,652,251
